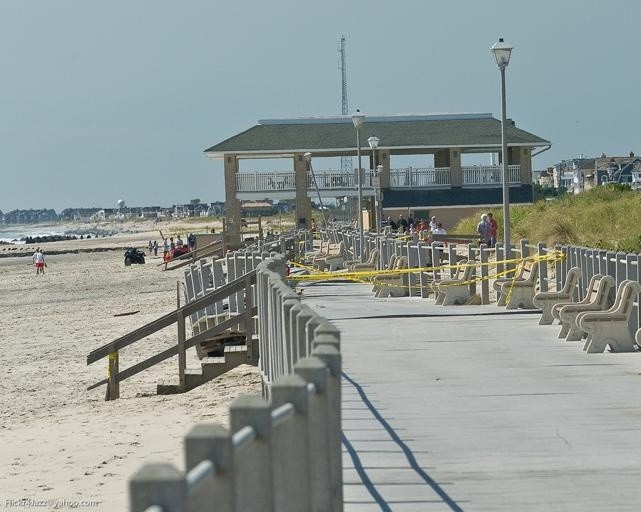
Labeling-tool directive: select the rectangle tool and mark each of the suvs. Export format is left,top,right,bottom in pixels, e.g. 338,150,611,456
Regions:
121,248,147,266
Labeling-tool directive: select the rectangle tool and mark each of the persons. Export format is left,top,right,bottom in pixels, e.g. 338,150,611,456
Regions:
477,214,491,248
211,226,215,233
311,218,317,239
487,213,497,248
380,212,448,252
32,248,42,271
36,249,45,274
148,234,183,261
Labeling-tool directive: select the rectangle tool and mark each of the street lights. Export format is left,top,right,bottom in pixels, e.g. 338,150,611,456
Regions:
486,25,520,291
348,108,382,258
303,151,334,243
